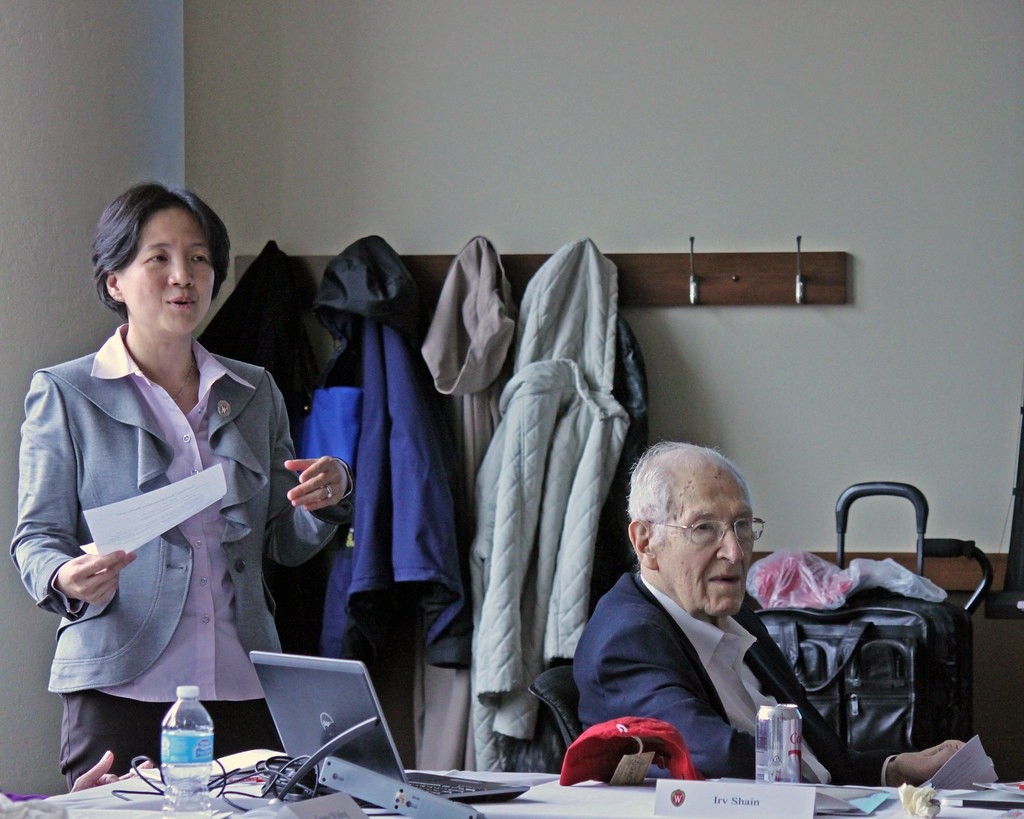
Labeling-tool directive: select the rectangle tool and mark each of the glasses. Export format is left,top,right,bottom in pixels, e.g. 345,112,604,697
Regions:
647,518,765,545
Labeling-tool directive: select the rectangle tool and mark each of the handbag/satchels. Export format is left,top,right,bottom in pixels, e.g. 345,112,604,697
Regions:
745,547,948,611
298,338,361,471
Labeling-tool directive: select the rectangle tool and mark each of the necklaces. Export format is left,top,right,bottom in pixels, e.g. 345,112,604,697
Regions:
170,357,196,404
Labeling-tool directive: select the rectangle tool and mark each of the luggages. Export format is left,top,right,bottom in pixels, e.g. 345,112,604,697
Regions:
753,482,975,752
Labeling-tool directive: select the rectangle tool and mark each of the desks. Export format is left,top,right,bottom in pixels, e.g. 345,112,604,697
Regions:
22,747,1024,819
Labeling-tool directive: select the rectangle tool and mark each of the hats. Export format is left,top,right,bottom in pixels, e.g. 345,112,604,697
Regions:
559,717,706,786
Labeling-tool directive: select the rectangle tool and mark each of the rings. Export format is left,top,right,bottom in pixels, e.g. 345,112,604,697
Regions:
326,484,334,498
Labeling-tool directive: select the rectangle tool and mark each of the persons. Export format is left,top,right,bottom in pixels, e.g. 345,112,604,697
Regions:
10,180,356,792
573,441,969,787
0,750,153,801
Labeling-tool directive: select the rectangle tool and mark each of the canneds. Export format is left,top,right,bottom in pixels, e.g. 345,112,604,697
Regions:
755,704,802,783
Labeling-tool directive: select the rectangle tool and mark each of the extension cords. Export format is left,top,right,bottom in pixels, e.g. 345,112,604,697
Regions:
318,755,485,818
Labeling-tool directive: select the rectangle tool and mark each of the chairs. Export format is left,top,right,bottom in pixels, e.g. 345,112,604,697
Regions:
527,664,583,748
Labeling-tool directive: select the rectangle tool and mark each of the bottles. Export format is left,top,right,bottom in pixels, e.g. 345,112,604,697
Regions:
160,685,215,819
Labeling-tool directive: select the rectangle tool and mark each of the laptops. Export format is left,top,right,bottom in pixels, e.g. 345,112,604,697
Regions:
248,650,530,816
